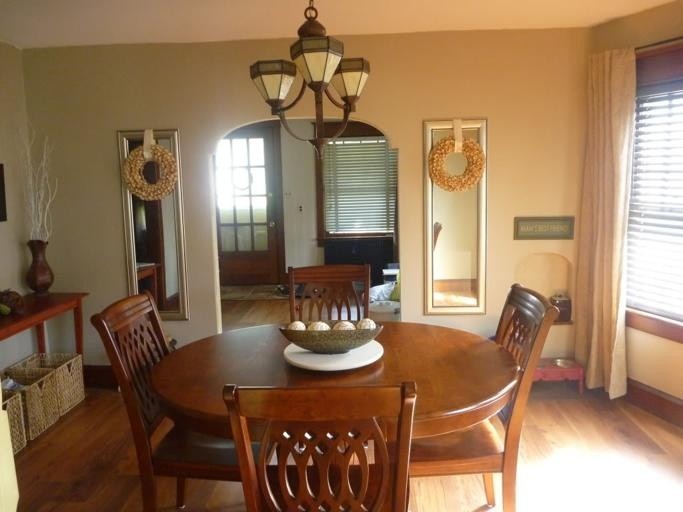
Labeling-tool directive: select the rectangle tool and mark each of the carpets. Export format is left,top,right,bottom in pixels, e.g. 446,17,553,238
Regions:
218,282,299,301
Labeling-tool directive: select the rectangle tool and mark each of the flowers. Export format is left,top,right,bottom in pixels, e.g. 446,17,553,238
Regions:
426,134,487,193
119,140,179,203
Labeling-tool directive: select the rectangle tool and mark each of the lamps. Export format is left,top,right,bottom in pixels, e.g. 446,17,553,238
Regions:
244,1,373,166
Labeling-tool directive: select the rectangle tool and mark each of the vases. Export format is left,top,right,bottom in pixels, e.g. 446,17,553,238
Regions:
23,238,54,301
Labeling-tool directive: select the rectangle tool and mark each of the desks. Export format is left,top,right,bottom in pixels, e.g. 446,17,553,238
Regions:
0,288,91,383
137,314,524,511
133,259,163,309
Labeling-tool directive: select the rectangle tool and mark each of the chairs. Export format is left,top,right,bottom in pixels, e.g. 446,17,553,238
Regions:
283,257,375,326
90,282,271,512
218,377,422,512
376,280,565,512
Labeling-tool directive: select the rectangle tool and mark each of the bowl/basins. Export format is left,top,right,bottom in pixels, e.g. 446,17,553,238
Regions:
552,358,578,370
278,320,383,356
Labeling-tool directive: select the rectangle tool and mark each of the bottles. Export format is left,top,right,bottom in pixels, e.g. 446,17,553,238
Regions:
552,294,571,322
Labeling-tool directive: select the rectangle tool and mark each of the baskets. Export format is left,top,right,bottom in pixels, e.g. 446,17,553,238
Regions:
0,351,87,455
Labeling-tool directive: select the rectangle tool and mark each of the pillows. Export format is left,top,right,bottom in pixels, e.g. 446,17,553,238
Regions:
360,280,393,302
389,268,402,301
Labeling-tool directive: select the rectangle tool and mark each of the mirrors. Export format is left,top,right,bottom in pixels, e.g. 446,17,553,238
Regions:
419,117,490,319
111,126,191,324
